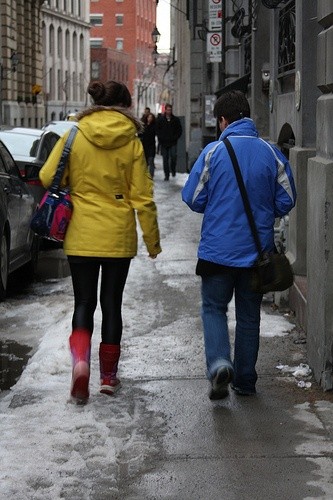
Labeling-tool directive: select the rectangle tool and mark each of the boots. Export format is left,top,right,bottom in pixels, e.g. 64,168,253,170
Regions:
98,343,123,394
67,327,92,399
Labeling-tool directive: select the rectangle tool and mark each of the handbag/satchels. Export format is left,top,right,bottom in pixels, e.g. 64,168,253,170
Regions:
32,184,73,250
251,247,294,294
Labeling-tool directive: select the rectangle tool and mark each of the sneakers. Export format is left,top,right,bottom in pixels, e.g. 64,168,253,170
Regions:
209,357,233,399
231,378,259,397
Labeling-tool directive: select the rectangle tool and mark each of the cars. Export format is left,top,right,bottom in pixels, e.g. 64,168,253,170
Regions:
0,112,82,299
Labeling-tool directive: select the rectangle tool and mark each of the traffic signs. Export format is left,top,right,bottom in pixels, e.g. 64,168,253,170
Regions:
209,17,223,31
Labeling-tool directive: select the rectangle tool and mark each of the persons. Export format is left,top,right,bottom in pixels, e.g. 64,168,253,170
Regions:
180,90,298,400
138,103,156,180
156,103,182,181
38,80,162,400
